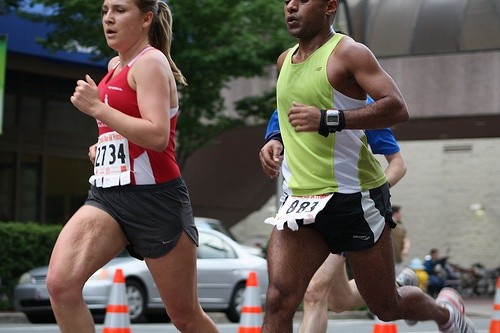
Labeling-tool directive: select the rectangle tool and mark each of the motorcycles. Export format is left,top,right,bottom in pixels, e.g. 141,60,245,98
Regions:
413,251,500,300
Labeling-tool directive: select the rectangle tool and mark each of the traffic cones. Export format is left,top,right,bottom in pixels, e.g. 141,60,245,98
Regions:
238,272,264,333
103,269,133,333
373,315,398,333
490,277,500,332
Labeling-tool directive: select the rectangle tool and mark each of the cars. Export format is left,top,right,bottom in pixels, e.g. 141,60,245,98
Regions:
16,216,270,323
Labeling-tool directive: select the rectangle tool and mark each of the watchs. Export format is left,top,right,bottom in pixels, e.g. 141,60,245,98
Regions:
325,108,340,133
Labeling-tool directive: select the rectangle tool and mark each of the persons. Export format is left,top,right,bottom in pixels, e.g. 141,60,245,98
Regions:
45,0,220,333
391,206,409,275
260,0,478,333
259,30,420,333
422,247,450,298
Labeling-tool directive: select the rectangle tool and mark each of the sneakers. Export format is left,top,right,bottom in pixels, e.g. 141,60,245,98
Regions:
436,286,475,333
395,268,421,326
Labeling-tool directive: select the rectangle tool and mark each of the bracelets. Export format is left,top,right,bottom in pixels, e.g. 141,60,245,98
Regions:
318,109,345,137
